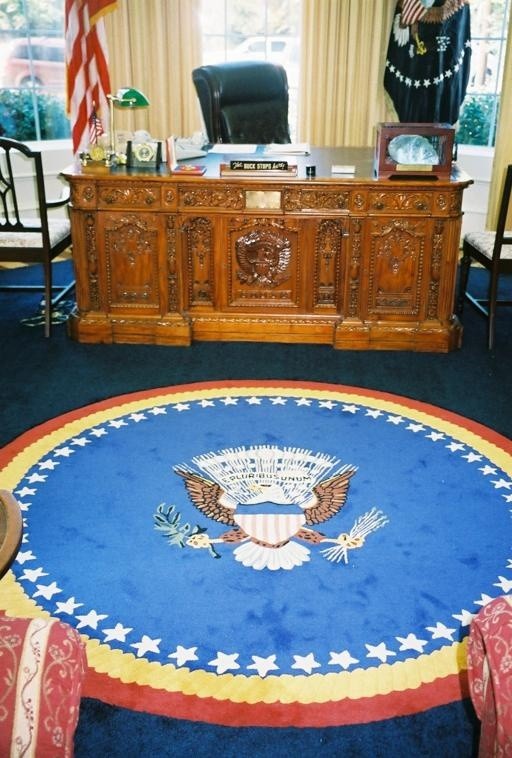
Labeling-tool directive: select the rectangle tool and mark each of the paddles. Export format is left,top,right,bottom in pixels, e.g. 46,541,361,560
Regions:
0,258,511,758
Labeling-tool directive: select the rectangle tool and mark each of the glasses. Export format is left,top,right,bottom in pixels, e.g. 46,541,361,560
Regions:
106,88,150,168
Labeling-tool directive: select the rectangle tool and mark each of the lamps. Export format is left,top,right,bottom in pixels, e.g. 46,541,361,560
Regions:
453,164,512,350
0,137,77,340
192,61,291,144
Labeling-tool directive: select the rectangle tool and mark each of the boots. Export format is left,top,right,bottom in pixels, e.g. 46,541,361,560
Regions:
0,36,67,102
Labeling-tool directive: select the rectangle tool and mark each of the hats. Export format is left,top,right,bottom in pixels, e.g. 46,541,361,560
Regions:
237,36,289,61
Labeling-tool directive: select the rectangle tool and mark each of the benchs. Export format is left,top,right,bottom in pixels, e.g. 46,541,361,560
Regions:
383,0,472,127
61,1,127,168
90,104,103,147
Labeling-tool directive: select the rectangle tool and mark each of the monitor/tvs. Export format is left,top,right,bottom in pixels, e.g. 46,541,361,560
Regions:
265,142,313,156
219,159,297,180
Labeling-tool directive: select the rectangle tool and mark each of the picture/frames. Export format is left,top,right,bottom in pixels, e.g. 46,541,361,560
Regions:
58,145,474,355
